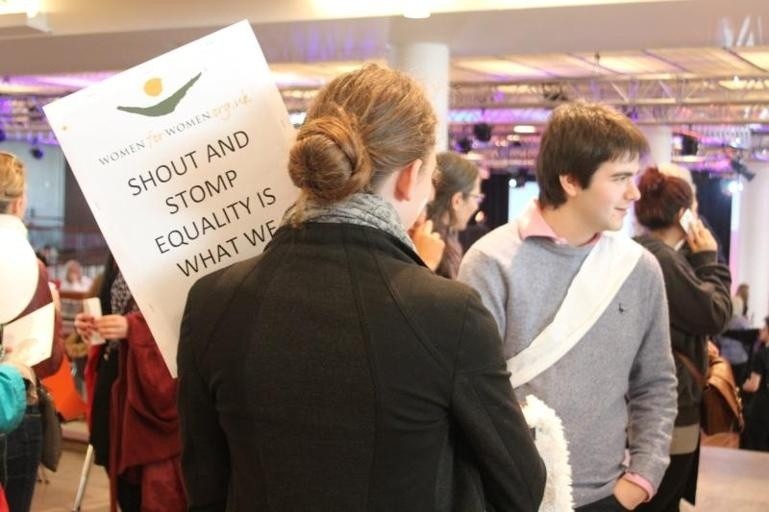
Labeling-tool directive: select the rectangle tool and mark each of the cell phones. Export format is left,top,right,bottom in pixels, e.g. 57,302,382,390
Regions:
679,208,696,234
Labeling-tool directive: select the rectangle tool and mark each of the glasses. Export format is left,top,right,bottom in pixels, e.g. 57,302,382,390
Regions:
469,192,485,206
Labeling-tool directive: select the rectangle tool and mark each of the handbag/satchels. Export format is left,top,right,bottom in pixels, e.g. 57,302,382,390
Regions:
699,356,745,437
37,386,62,472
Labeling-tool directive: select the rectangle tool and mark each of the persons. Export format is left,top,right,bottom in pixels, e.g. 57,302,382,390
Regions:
175,63,548,511
0,152,190,510
630,167,734,512
458,97,678,512
429,152,483,279
732,283,768,393
